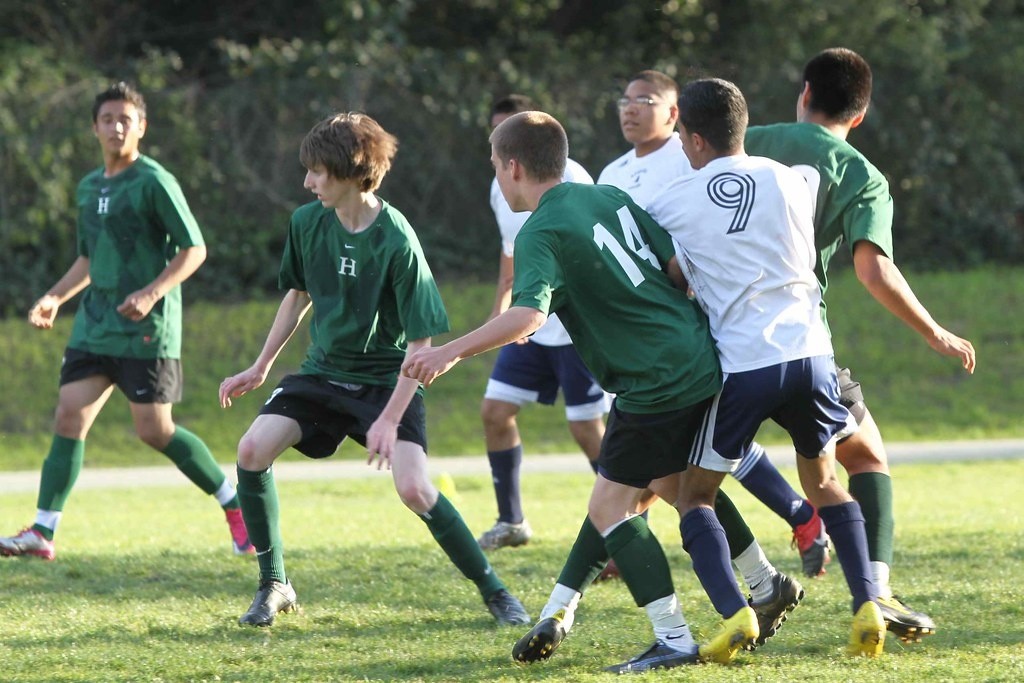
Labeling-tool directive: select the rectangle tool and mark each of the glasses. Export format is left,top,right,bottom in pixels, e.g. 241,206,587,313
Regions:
615,97,671,111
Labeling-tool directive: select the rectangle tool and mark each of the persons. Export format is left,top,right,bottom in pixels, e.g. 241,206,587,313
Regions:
477,95,648,549
400,109,803,672
595,71,832,578
0,79,256,557
512,46,975,660
645,78,888,663
219,112,530,628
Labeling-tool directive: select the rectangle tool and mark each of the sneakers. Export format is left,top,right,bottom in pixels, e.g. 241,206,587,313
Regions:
0,525,56,561
239,572,297,628
791,498,831,578
485,589,532,625
224,507,256,558
511,607,567,666
742,571,804,651
846,601,887,660
589,558,621,586
876,593,936,643
477,517,531,552
601,640,704,676
698,606,761,665
